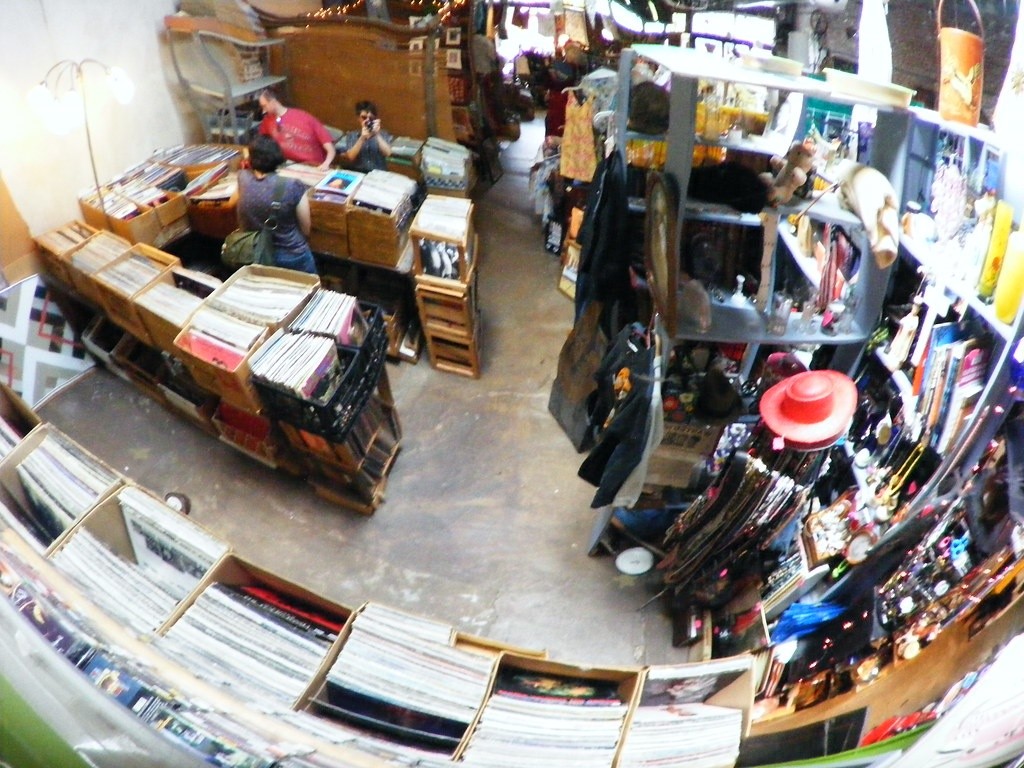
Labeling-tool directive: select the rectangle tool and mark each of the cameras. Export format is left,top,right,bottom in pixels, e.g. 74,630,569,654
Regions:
366,120,374,131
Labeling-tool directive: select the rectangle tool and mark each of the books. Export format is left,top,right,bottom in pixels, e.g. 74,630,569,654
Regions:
911,322,992,458
0,126,752,768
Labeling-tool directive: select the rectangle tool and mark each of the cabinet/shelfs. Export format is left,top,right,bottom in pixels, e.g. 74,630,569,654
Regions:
167,29,292,145
614,44,1024,542
412,198,481,378
270,365,404,513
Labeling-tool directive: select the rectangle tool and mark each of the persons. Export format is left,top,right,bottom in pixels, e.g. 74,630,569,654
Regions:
237,134,315,273
346,101,391,173
257,90,336,172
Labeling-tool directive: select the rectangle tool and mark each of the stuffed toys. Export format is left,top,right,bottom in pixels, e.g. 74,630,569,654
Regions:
759,137,816,206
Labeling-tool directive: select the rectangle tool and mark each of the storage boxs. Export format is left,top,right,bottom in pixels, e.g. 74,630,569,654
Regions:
0,386,772,767
28,136,475,461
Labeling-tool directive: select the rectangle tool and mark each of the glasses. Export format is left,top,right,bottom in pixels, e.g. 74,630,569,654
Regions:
360,115,375,120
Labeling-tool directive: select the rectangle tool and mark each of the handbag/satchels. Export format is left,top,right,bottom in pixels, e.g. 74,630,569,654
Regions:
220,226,274,268
